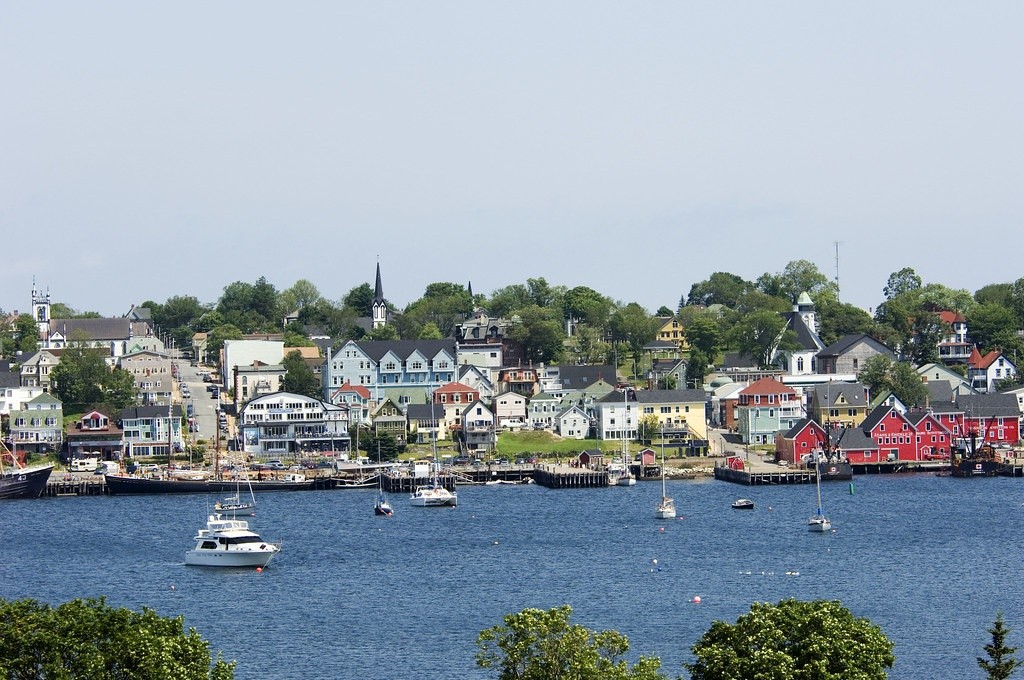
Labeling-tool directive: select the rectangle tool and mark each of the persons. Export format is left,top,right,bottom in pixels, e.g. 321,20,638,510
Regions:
558,459,575,468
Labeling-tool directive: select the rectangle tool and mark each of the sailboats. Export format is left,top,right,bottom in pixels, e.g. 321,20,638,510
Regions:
804,453,831,532
210,422,259,515
409,380,459,508
657,421,676,520
616,386,637,487
373,439,395,519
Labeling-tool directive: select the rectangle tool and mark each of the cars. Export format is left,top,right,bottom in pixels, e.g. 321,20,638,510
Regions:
141,464,158,472
94,467,102,474
172,352,230,434
426,456,537,465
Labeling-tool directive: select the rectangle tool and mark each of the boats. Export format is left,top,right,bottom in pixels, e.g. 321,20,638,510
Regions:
732,498,754,510
184,517,278,568
0,438,56,499
101,408,317,490
